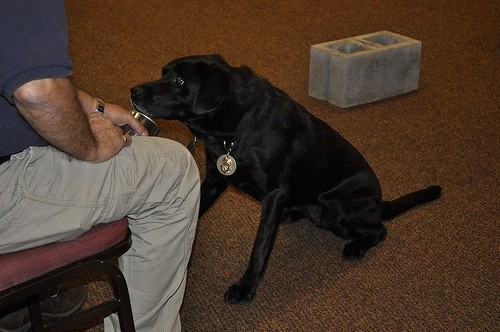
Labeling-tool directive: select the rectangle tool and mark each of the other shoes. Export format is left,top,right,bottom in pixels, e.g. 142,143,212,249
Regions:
0,286,87,332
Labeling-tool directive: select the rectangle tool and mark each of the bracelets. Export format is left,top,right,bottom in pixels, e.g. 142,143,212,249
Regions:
94,97,105,116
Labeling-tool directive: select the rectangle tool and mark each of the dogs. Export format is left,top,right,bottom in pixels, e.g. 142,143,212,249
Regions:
130,53,444,307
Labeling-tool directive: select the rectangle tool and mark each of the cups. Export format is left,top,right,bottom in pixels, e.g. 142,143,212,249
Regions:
121,112,163,139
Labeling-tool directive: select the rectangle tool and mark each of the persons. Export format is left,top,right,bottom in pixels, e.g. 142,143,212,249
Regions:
0,0,201,332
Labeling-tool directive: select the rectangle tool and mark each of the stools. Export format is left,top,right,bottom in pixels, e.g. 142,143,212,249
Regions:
0,215,137,332
